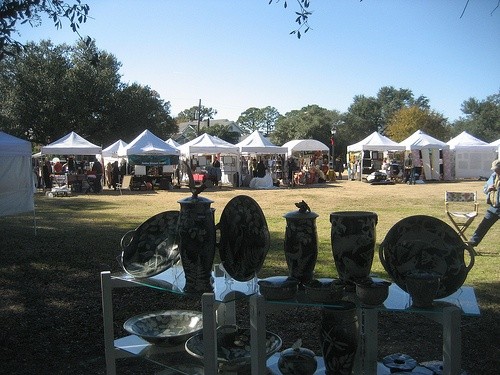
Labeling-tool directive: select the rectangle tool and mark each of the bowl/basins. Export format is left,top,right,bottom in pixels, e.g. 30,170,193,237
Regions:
215,196,271,282
185,323,283,365
302,281,349,303
123,310,204,345
257,278,300,300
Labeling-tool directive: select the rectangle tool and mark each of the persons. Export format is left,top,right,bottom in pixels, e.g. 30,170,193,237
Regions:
334,155,345,180
53,157,103,193
107,160,119,190
464,160,500,247
238,156,266,187
190,154,220,186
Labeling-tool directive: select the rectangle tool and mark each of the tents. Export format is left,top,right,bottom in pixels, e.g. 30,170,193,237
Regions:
31,152,74,173
40,131,106,193
95,139,130,170
446,130,500,180
346,130,406,182
164,137,180,150
399,129,452,181
281,139,330,183
117,129,181,189
235,130,288,187
177,132,241,188
0,130,37,236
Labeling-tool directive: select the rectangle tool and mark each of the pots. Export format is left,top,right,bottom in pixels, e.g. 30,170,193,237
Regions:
380,215,475,299
121,210,183,278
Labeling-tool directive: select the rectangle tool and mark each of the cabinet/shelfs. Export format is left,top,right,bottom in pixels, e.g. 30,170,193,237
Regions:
101,263,480,375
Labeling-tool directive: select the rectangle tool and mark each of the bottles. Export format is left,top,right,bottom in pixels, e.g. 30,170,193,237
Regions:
328,210,377,285
277,346,317,375
282,209,319,290
177,196,214,294
320,308,356,375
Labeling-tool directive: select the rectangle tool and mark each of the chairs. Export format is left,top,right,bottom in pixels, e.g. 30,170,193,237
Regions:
114,174,133,195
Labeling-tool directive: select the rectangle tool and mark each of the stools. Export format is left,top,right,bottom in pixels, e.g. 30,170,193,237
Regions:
85,175,98,195
445,190,479,256
